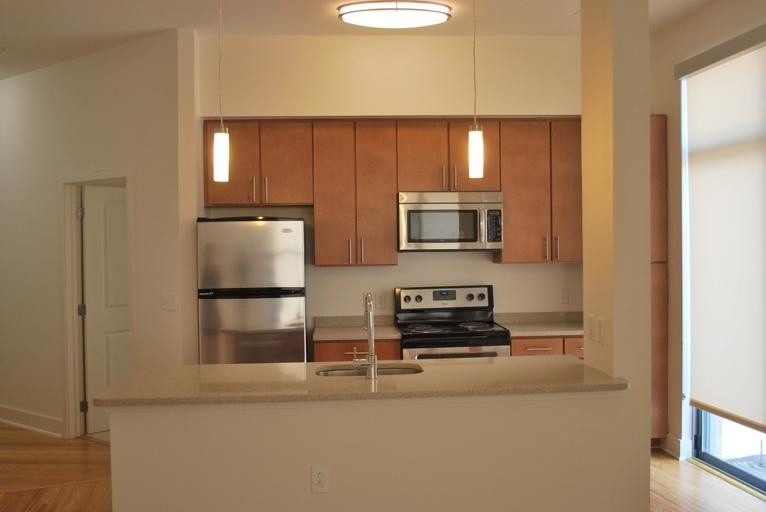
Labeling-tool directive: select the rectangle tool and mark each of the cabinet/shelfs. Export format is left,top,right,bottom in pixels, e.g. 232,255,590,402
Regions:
493,116,582,264
564,338,582,358
314,118,399,266
651,113,668,453
204,118,313,208
511,337,563,355
314,340,401,360
396,120,501,193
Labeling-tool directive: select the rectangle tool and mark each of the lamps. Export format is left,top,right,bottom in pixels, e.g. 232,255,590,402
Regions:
336,1,451,30
466,0,485,179
211,2,233,183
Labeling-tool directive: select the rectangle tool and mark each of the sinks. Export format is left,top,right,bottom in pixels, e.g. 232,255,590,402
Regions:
315,362,424,377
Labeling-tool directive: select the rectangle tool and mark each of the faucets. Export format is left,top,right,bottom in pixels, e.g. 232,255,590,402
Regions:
363,292,378,380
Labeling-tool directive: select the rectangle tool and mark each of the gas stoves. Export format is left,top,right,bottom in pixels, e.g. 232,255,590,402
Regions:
397,308,507,335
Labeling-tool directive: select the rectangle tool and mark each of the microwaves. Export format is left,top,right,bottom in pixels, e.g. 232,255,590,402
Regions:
396,190,504,253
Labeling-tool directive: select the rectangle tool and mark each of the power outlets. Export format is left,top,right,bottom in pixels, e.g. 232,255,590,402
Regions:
308,465,330,497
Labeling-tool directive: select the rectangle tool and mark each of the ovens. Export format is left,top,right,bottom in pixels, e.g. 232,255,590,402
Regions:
403,337,511,359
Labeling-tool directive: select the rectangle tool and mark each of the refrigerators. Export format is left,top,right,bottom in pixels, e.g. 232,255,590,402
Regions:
196,216,307,363
